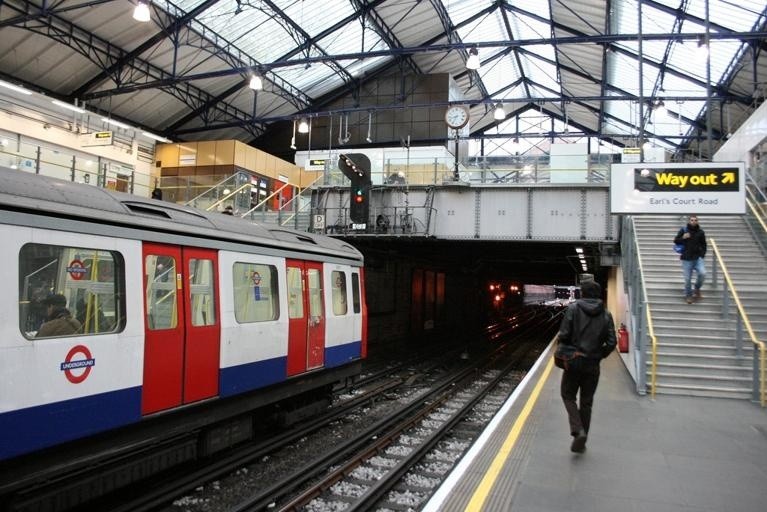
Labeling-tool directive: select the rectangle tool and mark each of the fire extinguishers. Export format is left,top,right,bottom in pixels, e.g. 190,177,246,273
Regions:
618,323,628,353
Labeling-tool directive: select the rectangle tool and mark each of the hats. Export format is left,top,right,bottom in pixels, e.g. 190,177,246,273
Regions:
582,281,601,297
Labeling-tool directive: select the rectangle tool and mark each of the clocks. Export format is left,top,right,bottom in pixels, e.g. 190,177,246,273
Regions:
444,102,470,128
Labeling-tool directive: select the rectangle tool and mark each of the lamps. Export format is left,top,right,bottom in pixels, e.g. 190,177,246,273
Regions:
521,99,686,146
298,118,312,136
248,69,262,89
492,101,505,119
466,47,480,69
287,106,375,149
130,0,151,21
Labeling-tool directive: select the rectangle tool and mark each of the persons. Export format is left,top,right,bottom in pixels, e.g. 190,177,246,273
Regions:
553,280,616,456
673,213,709,305
73,294,111,333
35,293,83,336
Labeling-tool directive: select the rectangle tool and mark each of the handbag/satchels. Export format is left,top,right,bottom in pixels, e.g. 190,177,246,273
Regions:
554,344,586,374
673,228,687,253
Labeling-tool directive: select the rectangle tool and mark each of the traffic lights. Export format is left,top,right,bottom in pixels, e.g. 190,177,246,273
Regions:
350,182,369,224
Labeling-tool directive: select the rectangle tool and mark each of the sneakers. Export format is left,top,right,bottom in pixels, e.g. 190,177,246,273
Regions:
571,430,586,452
686,287,700,303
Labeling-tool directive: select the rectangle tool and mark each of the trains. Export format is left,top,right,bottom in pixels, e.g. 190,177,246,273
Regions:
1,165,370,459
